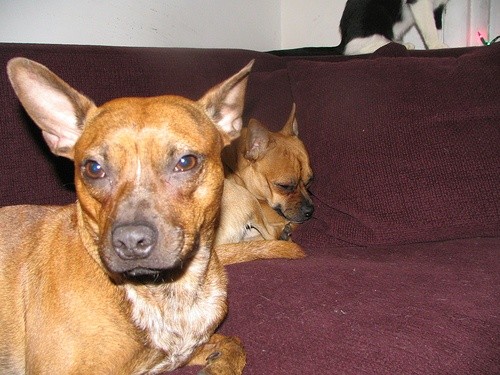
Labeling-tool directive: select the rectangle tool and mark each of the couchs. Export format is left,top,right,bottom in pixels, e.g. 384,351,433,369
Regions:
0,43,500,375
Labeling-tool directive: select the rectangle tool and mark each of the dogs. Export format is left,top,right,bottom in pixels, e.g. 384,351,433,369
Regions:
263,0,452,57
214,103,317,266
1,56,255,375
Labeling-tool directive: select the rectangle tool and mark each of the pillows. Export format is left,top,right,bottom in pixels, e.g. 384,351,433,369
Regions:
287,42,500,249
